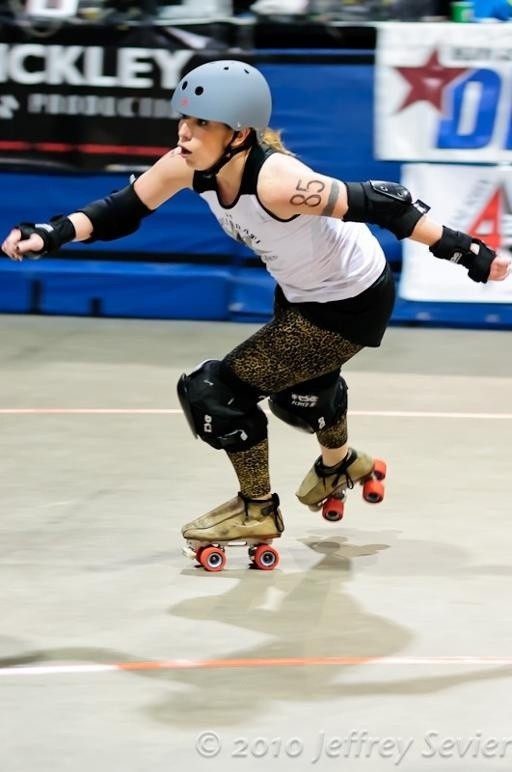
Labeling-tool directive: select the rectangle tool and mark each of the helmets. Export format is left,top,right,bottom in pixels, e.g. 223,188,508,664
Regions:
169,60,273,146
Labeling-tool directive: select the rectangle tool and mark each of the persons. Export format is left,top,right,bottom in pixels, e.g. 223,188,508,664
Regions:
1,55,512,541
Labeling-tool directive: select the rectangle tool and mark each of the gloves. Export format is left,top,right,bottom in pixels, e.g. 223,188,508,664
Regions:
427,224,499,285
12,213,77,262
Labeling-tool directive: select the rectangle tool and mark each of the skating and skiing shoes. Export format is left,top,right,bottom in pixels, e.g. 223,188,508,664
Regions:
181,492,284,572
296,447,386,521
